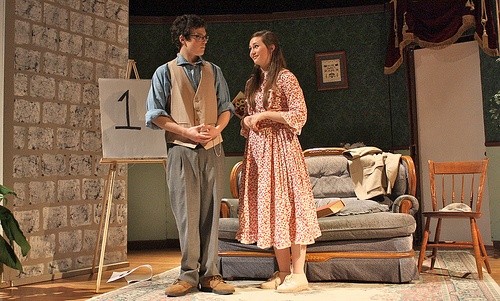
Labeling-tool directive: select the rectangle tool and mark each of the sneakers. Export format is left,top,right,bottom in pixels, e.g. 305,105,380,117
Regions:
276,272,309,293
260,270,290,289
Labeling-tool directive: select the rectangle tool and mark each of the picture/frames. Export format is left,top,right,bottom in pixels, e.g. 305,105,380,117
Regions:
316,52,349,90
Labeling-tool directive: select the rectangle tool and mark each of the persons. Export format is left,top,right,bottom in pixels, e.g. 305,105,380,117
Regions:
236,30,322,292
144,15,236,296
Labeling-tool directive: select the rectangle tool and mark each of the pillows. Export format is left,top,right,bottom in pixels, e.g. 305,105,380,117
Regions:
336,198,390,217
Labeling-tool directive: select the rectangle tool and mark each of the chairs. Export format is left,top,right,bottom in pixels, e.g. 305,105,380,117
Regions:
418,158,491,280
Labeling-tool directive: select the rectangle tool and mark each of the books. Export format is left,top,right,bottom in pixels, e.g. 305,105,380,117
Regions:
316,200,345,218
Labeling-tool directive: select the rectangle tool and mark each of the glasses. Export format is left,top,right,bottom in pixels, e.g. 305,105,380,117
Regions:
185,34,210,42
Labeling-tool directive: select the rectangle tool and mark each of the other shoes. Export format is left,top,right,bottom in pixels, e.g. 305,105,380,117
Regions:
164,278,199,297
198,275,235,294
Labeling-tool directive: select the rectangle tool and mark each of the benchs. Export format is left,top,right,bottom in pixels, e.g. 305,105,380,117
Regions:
219,214,416,283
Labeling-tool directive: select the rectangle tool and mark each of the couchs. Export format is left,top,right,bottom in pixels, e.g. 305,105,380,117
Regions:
218,147,418,218
